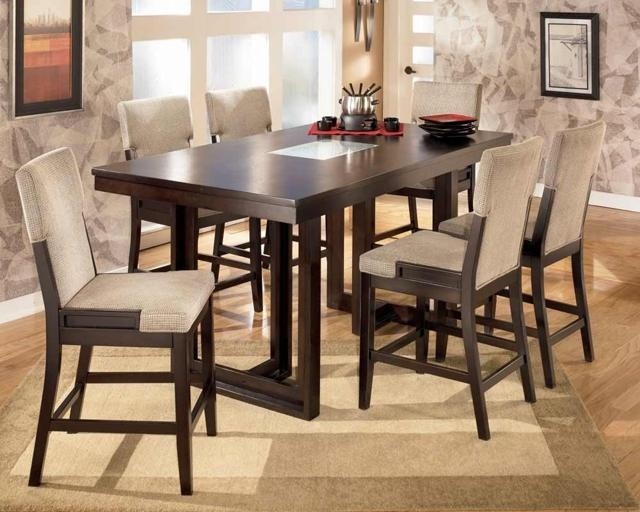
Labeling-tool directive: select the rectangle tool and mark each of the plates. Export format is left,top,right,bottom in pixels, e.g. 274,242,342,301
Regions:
418,113,478,138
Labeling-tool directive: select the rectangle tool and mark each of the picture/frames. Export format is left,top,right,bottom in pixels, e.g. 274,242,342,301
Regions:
539,11,600,100
8,0,85,120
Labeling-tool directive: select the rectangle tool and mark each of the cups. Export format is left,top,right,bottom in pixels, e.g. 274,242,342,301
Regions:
384,118,399,132
317,121,333,131
323,116,337,127
360,118,377,130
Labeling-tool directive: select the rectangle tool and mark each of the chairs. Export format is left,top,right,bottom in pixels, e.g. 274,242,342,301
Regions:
114,92,265,314
356,133,546,441
204,85,273,289
434,120,608,391
13,144,219,497
375,77,483,233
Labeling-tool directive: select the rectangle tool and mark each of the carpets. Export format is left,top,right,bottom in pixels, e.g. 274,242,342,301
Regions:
0,325,639,512
